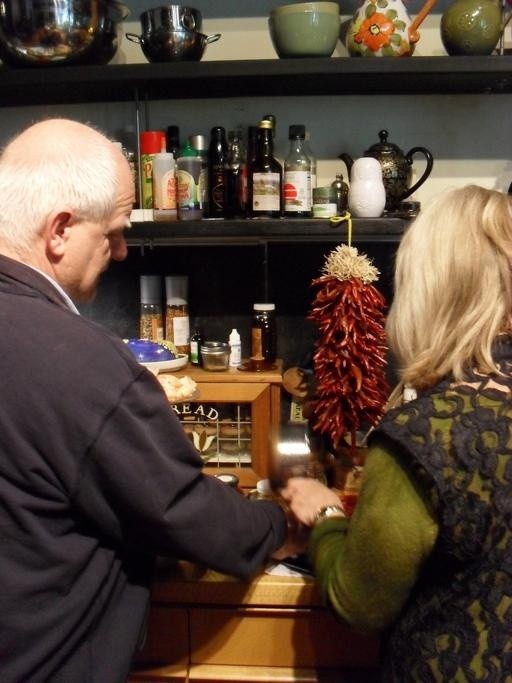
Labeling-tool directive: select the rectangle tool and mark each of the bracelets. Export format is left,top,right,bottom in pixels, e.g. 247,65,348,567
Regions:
307,502,349,529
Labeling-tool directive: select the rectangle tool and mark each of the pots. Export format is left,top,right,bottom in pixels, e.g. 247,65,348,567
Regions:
124,31,218,61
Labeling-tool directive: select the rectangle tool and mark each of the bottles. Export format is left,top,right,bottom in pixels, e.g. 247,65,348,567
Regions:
250,302,280,367
340,0,510,58
331,173,349,211
139,271,191,355
124,114,317,217
228,329,242,368
189,317,207,366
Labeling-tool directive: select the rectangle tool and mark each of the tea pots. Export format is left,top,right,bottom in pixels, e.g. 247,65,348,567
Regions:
337,126,436,211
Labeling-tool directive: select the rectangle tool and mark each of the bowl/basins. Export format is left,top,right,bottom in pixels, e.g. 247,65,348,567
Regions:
0,0,131,64
269,2,340,59
139,4,203,35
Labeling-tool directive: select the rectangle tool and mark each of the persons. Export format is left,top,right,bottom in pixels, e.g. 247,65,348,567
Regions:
280,184,510,680
2,118,307,680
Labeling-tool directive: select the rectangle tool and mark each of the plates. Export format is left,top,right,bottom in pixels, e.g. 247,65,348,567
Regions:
137,353,189,376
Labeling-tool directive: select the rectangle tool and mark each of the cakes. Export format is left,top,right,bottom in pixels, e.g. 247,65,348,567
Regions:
123,338,178,362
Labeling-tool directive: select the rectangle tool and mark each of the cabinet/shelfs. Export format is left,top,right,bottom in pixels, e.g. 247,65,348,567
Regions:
131,580,189,680
192,584,386,679
0,49,512,243
156,358,284,501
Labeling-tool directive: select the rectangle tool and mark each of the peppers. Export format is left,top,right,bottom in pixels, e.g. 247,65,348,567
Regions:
306,276,392,451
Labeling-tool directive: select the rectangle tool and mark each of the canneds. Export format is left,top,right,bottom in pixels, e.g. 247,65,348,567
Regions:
215,473,244,497
182,417,251,458
